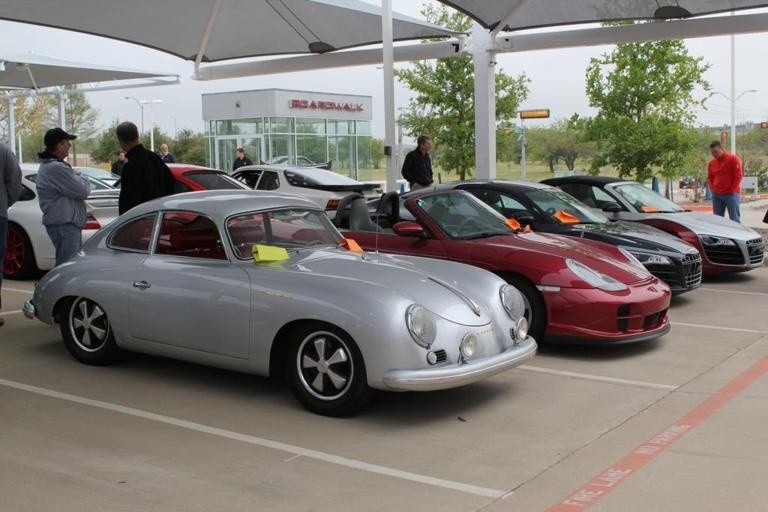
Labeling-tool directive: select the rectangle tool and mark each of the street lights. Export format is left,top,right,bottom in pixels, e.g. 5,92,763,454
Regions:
124,95,164,134
709,88,758,157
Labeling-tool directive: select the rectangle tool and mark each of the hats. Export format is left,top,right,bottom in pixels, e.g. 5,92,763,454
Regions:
43,127,78,148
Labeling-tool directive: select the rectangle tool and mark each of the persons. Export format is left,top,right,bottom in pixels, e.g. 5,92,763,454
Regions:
34,127,92,268
0,143,24,320
117,121,176,224
401,137,434,192
111,151,125,176
158,143,175,163
708,140,742,222
233,148,251,172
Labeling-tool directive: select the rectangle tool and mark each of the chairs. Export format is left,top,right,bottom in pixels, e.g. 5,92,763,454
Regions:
349,197,398,240
231,225,266,259
164,227,225,259
